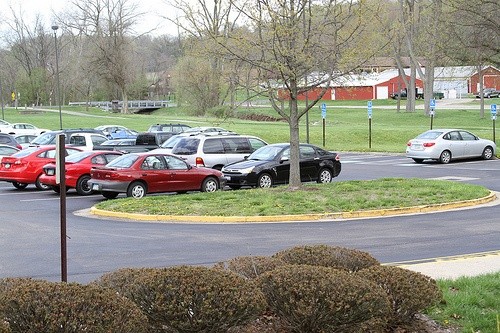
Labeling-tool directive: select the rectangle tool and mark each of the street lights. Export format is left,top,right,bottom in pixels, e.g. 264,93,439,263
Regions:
51,25,63,129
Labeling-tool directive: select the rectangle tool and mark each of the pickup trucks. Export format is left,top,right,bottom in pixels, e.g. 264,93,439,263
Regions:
390,88,425,99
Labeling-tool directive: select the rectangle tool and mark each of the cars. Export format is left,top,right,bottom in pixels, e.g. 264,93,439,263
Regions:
476,88,500,99
220,143,342,189
405,128,496,164
0,118,231,160
0,145,83,191
37,149,125,195
85,151,226,200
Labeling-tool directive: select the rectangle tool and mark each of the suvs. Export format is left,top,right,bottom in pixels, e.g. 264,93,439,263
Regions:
169,130,271,172
